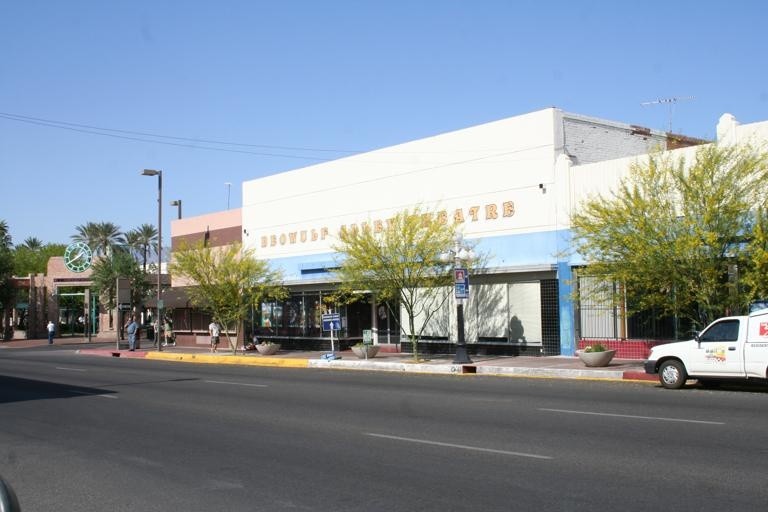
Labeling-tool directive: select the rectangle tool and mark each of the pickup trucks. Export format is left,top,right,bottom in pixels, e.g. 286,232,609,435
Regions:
644,307,768,389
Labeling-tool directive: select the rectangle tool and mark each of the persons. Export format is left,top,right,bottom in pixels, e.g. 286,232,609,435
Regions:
207,316,221,354
125,317,139,352
77,314,83,323
152,319,158,348
162,318,176,347
46,320,55,344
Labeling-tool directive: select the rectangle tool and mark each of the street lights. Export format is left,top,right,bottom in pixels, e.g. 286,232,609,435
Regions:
140,167,163,354
169,198,182,220
223,180,234,210
440,239,477,343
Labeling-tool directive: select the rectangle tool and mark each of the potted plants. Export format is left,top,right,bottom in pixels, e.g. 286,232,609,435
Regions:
351,342,380,359
255,341,282,355
576,344,617,367
